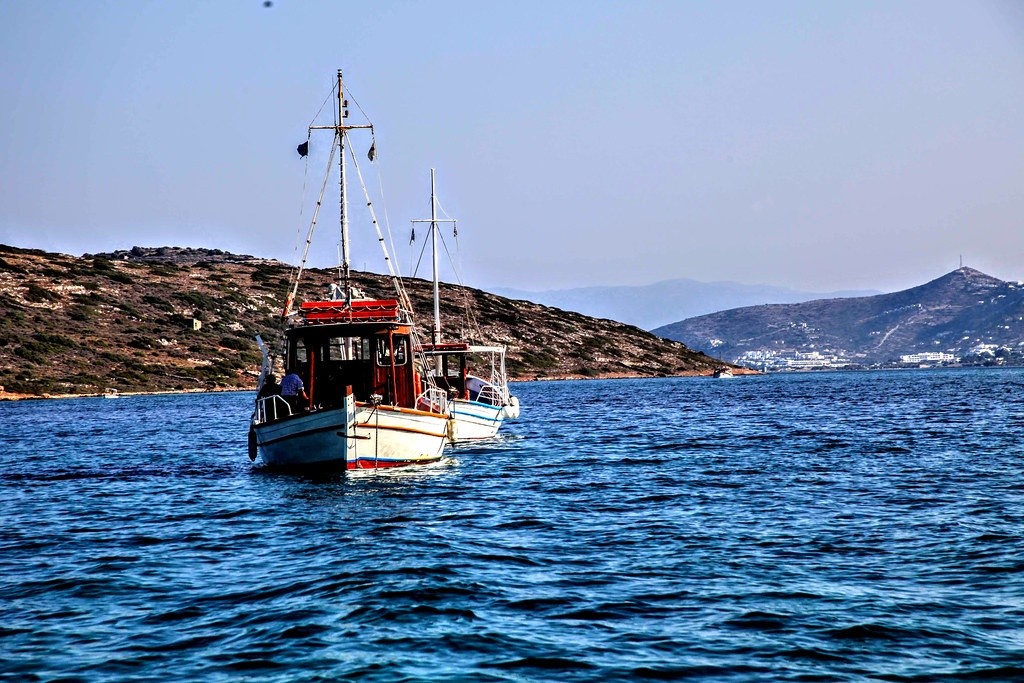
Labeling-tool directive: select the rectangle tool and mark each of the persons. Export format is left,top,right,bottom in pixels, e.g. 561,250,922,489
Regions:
258,369,309,419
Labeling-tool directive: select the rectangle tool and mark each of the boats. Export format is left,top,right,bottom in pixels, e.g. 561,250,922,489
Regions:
712,351,734,378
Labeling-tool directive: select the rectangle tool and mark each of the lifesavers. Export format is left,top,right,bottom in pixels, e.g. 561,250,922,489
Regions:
415,371,424,405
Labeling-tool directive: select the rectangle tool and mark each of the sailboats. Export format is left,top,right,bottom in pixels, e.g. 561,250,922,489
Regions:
402,167,511,448
249,65,450,480
